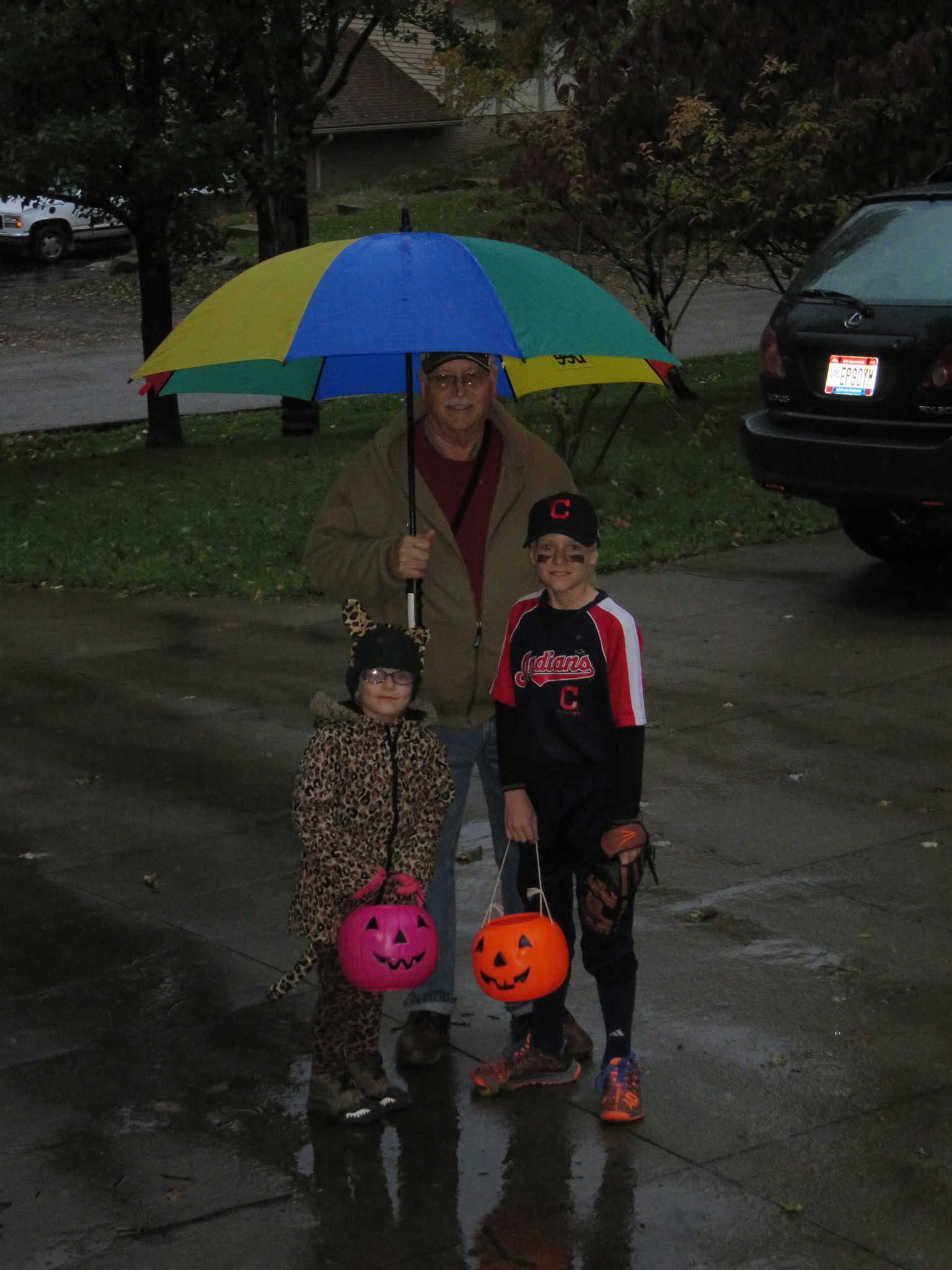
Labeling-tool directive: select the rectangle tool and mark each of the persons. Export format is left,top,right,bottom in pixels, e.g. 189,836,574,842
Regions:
268,595,454,1127
471,491,656,1127
301,350,595,1069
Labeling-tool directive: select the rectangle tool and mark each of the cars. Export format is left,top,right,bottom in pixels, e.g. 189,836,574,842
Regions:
0,165,237,267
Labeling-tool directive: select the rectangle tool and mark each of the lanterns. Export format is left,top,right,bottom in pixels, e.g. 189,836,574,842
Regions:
470,841,569,1002
337,881,439,994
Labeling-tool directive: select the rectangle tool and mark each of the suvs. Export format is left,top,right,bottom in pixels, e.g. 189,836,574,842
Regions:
740,170,952,563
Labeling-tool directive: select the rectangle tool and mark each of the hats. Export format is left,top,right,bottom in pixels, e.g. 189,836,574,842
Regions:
523,491,601,550
418,351,493,381
342,597,431,698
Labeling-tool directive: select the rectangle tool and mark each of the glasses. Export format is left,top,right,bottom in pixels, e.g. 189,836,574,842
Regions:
359,667,415,685
427,372,489,392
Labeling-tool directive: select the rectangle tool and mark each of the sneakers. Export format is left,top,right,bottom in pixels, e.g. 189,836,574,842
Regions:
346,1053,411,1112
306,1067,384,1125
510,1005,593,1060
595,1052,643,1122
396,1008,452,1068
470,1025,581,1090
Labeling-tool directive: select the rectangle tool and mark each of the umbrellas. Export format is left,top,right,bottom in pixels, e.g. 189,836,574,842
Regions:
124,205,683,629
